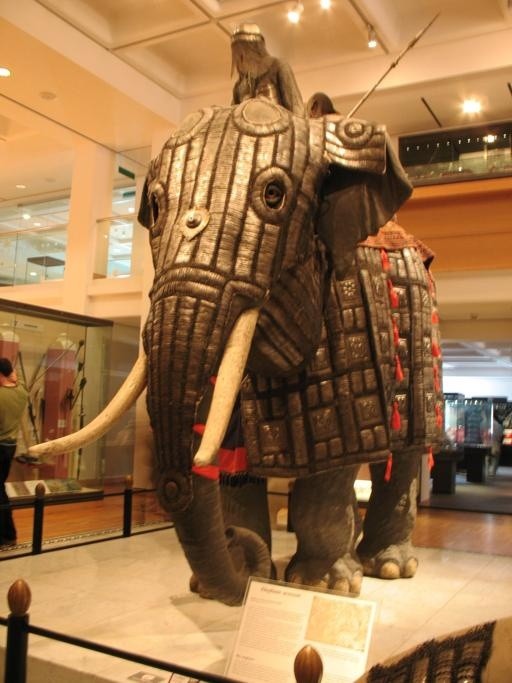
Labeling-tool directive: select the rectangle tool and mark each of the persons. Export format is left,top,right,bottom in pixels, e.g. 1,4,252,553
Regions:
306,89,336,118
485,410,504,477
229,16,305,117
0,358,30,547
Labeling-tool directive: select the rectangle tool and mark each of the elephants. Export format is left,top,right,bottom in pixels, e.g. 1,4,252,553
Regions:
20,93,443,608
